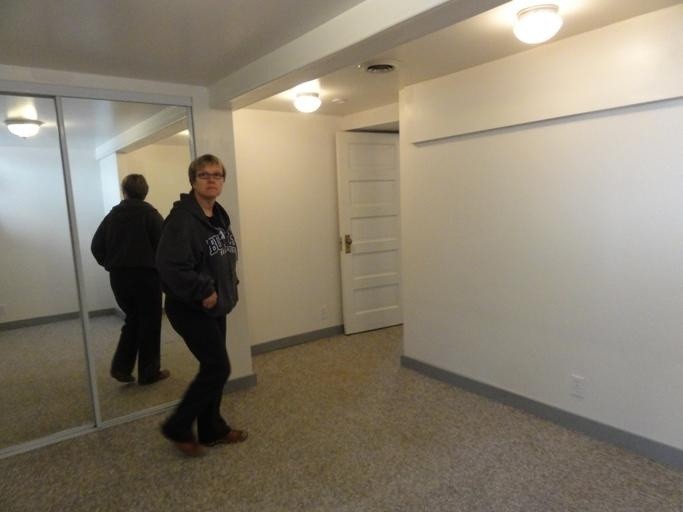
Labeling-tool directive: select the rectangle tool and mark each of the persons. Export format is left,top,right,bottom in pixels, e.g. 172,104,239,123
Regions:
90,174,171,385
154,154,248,459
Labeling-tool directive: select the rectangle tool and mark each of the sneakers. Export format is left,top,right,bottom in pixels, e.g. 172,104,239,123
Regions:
159,423,248,457
109,369,169,386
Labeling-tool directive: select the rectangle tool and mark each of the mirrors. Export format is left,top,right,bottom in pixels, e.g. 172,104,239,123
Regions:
0,82,201,460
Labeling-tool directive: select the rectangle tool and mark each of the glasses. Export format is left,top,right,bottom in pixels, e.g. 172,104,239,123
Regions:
195,172,224,179
